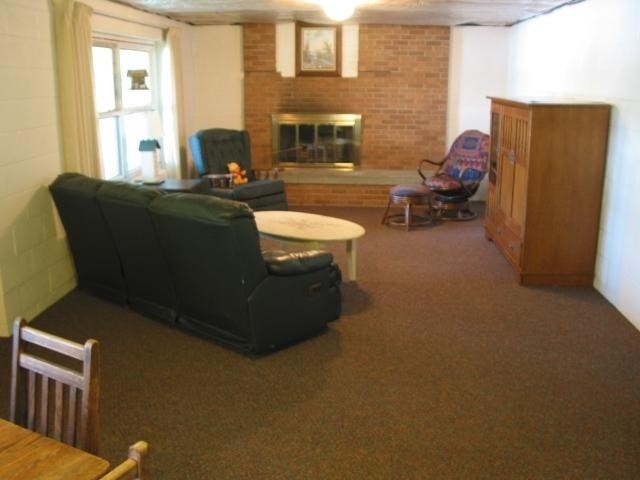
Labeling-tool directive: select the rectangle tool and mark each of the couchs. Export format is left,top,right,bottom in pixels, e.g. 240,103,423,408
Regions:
47,171,342,361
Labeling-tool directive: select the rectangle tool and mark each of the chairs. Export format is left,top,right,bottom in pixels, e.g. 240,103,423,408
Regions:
7,316,102,457
187,127,287,212
417,129,490,221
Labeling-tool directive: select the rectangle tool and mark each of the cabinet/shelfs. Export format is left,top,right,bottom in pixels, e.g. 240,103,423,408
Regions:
485,100,610,289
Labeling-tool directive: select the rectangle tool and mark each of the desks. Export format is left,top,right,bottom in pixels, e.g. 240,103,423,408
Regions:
0,420,147,479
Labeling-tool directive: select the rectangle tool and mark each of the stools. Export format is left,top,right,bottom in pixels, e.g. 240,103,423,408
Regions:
382,185,436,231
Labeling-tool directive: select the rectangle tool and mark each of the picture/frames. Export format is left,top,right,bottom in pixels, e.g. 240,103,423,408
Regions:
296,20,341,78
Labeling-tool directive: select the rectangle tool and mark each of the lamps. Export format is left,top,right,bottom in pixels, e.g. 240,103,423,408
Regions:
139,140,167,186
